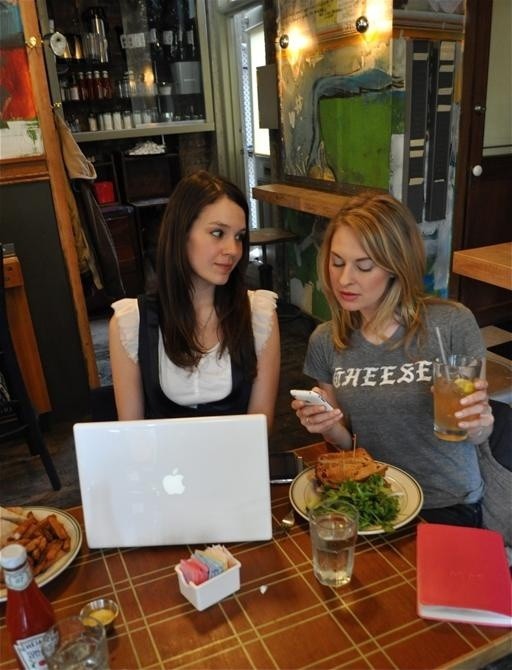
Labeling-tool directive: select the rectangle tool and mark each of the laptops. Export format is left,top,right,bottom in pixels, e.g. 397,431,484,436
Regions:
72,413,273,550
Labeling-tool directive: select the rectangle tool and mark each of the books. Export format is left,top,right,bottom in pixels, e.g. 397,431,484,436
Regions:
413,521,511,628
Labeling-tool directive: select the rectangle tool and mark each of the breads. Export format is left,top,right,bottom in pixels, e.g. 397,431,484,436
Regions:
315,447,389,490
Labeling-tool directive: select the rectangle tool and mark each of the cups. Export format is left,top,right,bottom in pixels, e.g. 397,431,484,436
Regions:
40,613,115,670
78,597,121,634
429,353,483,442
308,498,361,588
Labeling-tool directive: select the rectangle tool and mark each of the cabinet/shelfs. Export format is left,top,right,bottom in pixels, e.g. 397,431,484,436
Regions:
49,26,214,141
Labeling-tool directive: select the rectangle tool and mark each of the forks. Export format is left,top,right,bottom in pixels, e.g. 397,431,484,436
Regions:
277,507,297,531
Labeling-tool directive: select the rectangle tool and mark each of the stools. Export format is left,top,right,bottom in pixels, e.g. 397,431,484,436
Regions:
244,226,316,335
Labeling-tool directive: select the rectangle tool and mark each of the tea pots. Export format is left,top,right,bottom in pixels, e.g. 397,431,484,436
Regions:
77,30,108,65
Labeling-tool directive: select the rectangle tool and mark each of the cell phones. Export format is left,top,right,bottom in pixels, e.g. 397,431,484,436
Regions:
269,450,299,485
289,389,335,413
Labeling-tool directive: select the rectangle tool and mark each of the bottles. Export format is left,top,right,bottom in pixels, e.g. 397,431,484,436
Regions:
0,540,63,670
88,8,110,63
86,103,158,132
58,65,139,101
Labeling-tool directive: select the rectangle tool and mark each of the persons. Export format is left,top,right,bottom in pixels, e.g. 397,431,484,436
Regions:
288,191,497,540
107,168,283,466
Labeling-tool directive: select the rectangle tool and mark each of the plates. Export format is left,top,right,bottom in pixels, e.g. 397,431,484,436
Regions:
0,502,84,603
287,460,427,536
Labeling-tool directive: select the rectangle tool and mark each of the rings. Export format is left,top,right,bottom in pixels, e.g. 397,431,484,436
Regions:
306,417,310,425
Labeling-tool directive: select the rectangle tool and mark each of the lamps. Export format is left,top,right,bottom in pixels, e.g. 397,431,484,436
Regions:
355,16,370,35
278,31,289,48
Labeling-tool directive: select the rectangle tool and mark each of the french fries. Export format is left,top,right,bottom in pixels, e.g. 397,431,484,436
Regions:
0,512,71,590
